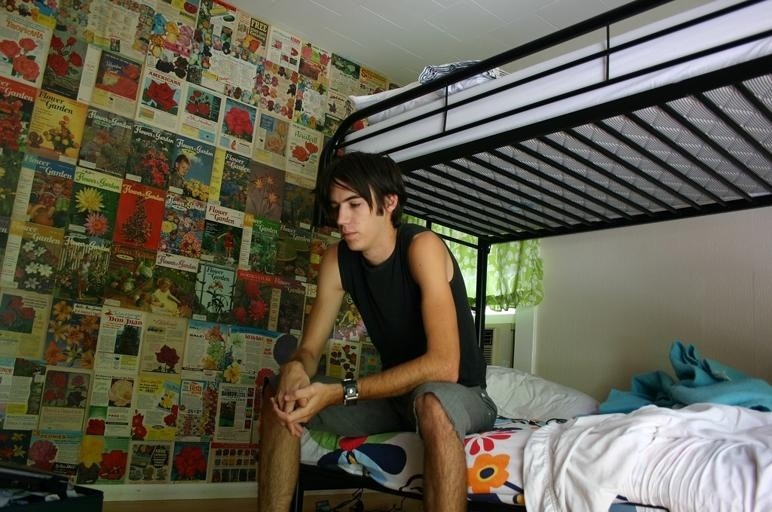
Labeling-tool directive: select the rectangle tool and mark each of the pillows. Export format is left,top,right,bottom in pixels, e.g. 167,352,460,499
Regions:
348,69,440,126
482,360,600,420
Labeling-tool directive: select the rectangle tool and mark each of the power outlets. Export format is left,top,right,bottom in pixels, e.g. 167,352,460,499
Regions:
316,500,331,512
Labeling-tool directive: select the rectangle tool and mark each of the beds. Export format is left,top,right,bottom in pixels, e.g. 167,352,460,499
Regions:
312,1,772,246
289,341,771,512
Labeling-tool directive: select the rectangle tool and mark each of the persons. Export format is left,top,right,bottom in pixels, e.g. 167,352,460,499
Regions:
32,177,68,229
67,246,96,301
215,227,240,257
257,152,497,512
151,270,181,313
169,152,192,193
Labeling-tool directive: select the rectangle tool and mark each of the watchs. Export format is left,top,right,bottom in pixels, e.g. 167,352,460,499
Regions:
341,378,359,408
